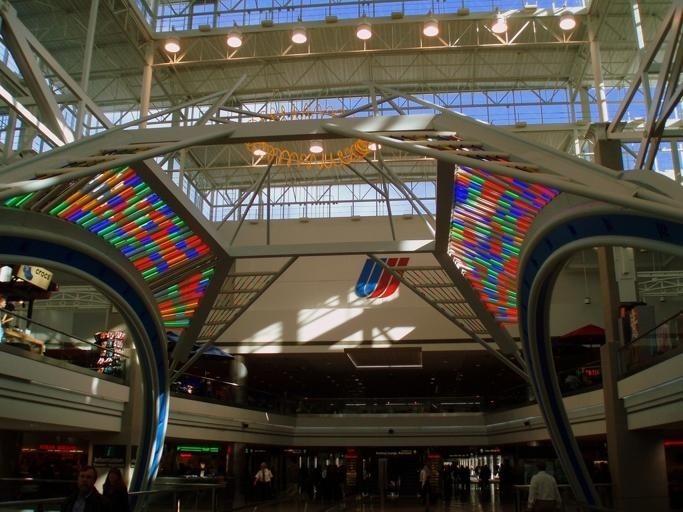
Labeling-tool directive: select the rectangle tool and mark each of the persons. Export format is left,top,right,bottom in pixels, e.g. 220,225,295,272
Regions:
61,466,113,512
0,296,15,343
103,468,129,512
417,456,513,506
252,461,274,512
301,462,346,505
597,462,608,508
526,461,562,512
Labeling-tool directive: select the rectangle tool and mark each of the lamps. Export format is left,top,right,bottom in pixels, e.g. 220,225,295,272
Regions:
164,31,181,53
226,25,243,48
557,8,577,32
489,8,509,34
291,21,308,45
309,140,324,154
355,8,372,41
422,8,439,38
